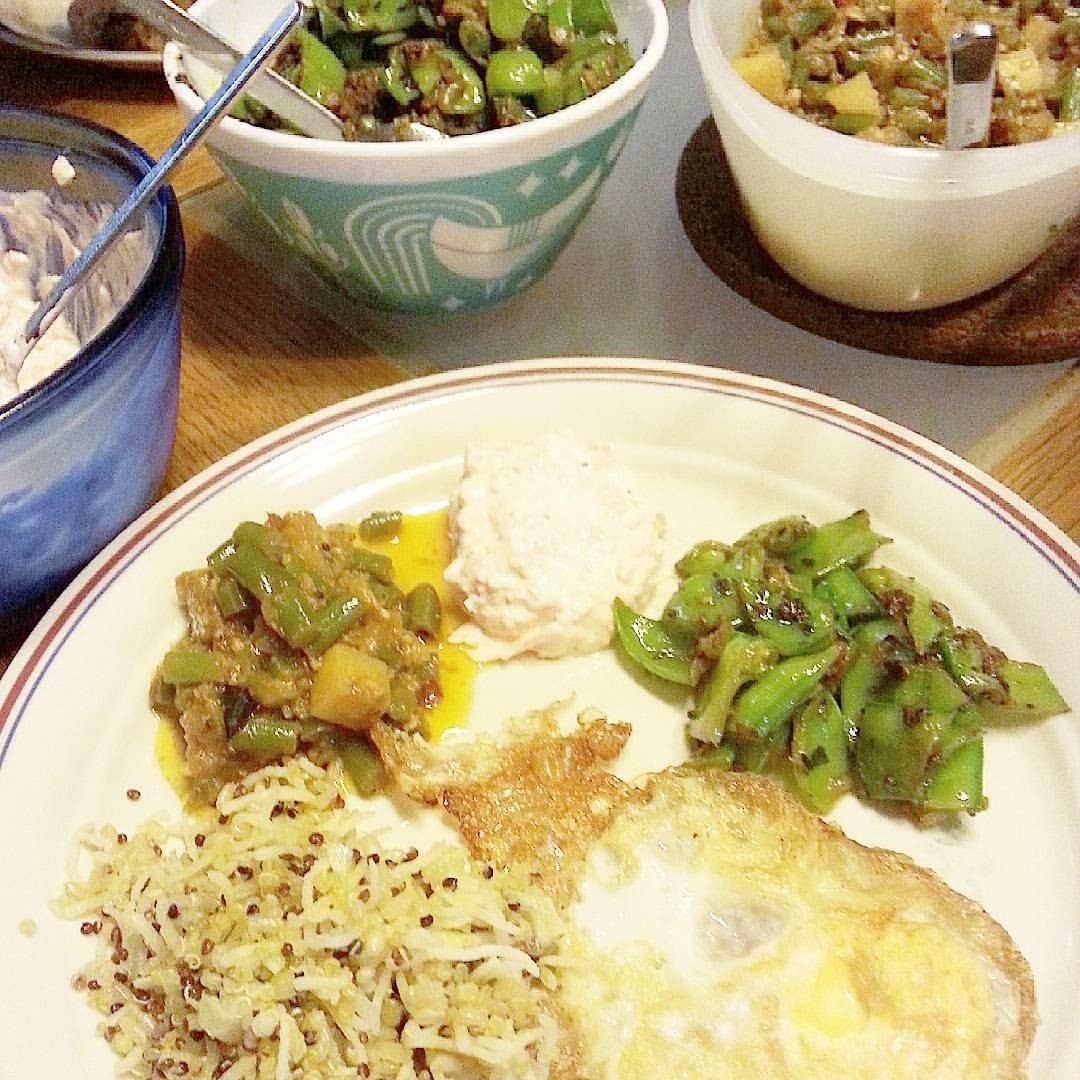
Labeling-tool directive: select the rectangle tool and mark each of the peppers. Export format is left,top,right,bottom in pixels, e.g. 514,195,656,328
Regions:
242,4,629,135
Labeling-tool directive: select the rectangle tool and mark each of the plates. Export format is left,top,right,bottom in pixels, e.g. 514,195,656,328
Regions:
0,0,164,73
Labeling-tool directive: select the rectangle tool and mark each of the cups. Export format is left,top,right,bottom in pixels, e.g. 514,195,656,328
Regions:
1,356,1080,1079
687,0,1080,311
0,104,183,617
160,0,669,314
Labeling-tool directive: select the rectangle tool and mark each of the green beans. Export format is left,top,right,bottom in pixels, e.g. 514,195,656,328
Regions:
150,498,449,802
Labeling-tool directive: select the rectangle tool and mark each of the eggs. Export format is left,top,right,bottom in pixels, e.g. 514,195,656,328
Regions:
375,699,1039,1079
